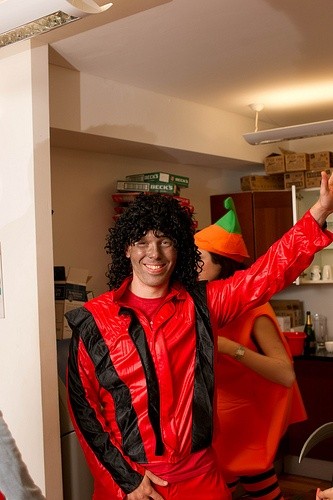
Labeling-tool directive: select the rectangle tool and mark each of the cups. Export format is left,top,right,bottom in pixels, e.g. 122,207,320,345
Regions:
325,341,333,352
322,264,332,280
311,265,321,280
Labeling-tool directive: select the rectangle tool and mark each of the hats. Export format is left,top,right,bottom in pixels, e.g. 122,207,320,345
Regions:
193,196,250,264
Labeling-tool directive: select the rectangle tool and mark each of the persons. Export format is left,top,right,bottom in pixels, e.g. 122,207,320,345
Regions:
64,170,333,500
316,486,333,500
193,197,308,500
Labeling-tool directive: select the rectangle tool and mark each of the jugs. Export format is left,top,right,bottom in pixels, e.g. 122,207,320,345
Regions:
312,312,328,354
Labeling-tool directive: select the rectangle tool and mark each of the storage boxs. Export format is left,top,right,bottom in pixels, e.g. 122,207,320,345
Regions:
240,150,333,191
283,330,308,356
52,265,97,340
111,171,198,229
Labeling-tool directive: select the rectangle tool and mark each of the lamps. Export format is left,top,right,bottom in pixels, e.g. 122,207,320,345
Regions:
0,0,114,48
241,103,333,145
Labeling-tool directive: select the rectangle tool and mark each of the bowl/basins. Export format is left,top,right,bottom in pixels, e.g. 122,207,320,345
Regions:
282,332,307,356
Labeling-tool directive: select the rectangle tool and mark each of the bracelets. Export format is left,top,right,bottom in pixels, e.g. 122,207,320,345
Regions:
233,345,245,361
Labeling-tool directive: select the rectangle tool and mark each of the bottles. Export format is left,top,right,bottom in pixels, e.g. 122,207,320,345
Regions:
304,311,316,354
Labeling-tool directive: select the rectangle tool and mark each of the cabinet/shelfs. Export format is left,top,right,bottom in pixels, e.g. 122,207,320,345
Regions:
292,184,333,285
289,358,333,461
209,190,296,285
